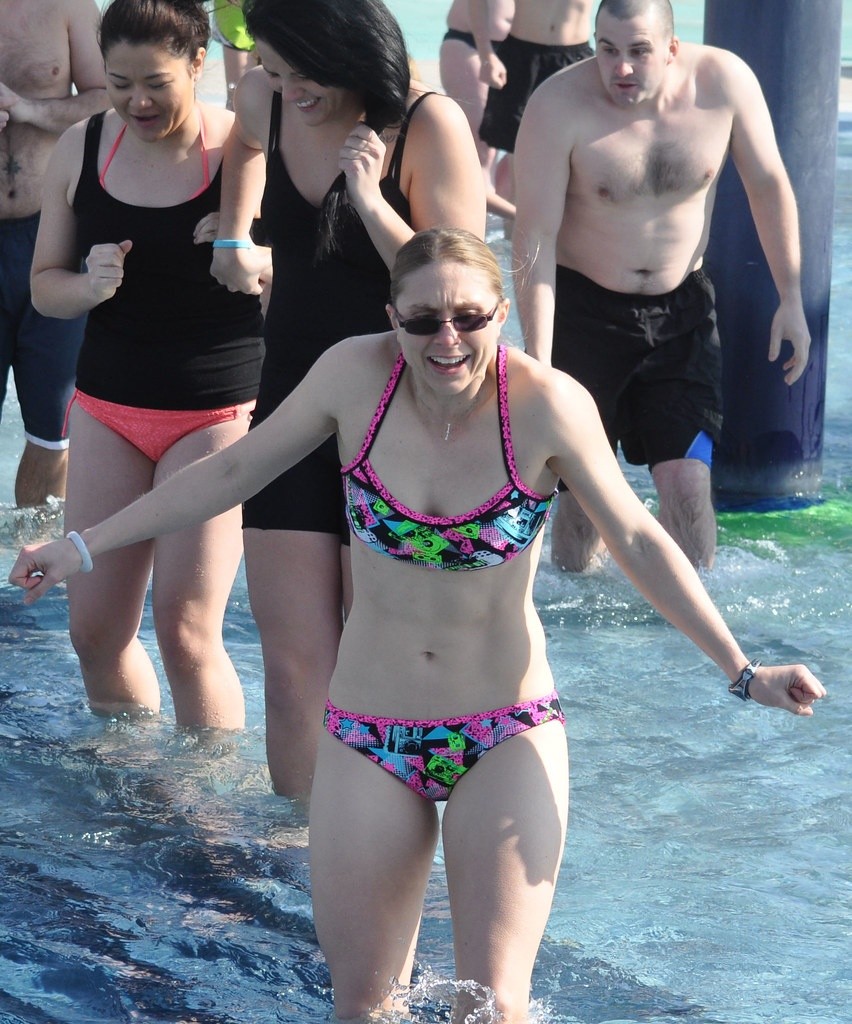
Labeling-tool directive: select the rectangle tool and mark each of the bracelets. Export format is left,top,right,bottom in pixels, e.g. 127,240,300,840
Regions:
66,530,94,574
728,657,763,702
212,239,255,249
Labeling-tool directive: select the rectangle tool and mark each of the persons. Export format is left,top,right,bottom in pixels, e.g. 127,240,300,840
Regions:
510,1,815,582
439,1,599,245
1,0,104,536
9,225,828,1022
26,0,274,733
208,0,486,850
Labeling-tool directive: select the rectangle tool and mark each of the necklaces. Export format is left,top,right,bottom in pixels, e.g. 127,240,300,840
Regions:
444,422,451,442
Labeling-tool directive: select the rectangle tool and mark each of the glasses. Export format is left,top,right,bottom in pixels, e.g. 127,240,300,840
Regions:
395,302,499,336
